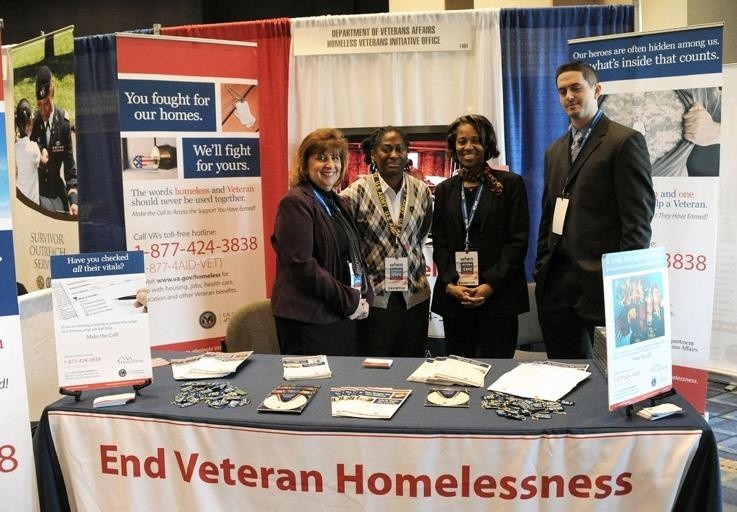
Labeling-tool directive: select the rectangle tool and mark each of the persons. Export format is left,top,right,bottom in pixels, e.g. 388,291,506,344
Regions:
643,298,658,340
271,127,374,357
29,65,81,220
531,60,654,361
649,283,664,337
675,86,718,178
629,301,649,344
337,124,434,355
428,116,532,358
12,99,50,207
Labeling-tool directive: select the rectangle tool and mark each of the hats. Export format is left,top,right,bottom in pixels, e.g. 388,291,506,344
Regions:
36,66,52,99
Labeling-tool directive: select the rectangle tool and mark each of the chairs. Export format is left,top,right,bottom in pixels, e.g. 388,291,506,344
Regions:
220,298,280,354
512,282,547,361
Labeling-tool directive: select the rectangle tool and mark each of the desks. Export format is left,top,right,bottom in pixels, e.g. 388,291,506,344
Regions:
43,352,705,512
